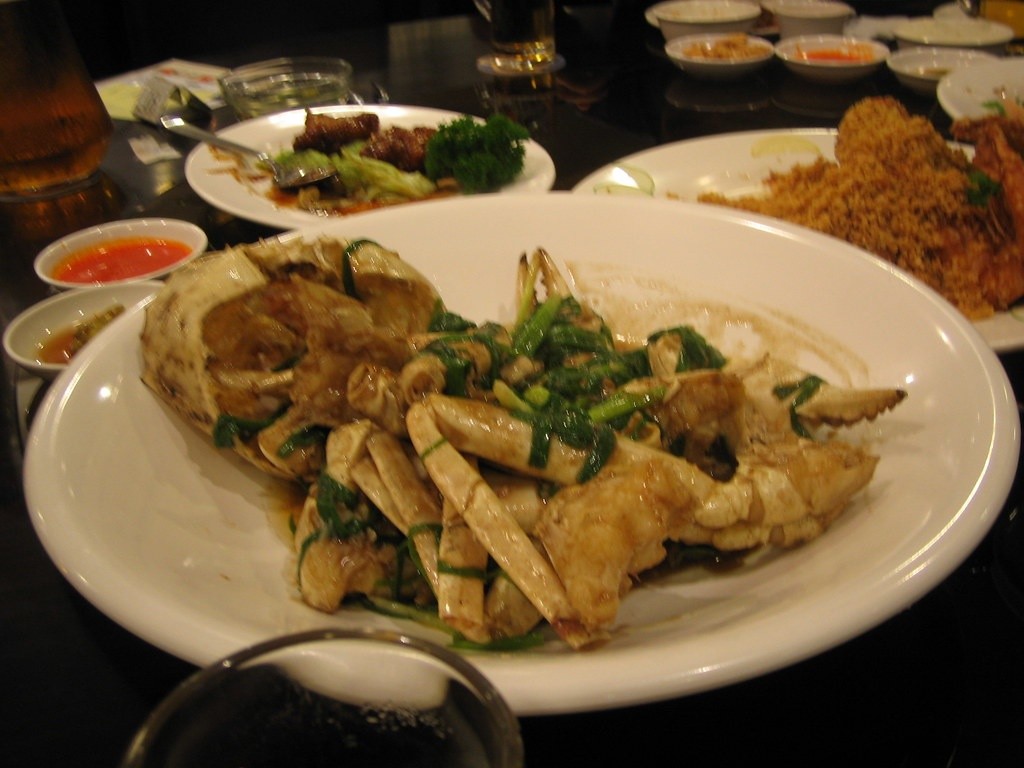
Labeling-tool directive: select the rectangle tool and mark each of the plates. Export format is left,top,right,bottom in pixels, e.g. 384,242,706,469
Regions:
571,125,1024,354
936,57,1024,124
3,281,165,382
892,17,1014,51
775,34,888,81
885,48,1000,93
185,104,556,228
664,33,774,80
21,190,1020,717
32,217,209,288
646,0,856,36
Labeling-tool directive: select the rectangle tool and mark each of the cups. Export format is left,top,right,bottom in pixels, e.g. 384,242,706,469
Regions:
0,0,114,199
215,55,353,123
119,628,526,768
489,0,555,71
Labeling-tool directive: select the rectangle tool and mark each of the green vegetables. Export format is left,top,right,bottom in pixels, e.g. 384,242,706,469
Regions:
244,104,530,201
216,254,831,650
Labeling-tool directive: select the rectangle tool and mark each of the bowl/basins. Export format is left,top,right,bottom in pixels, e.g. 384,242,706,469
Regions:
653,0,762,42
761,0,854,40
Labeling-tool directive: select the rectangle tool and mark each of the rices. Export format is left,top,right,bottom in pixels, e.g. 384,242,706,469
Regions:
689,96,1023,320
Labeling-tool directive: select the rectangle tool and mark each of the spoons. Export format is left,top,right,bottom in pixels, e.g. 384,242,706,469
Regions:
160,114,340,190
